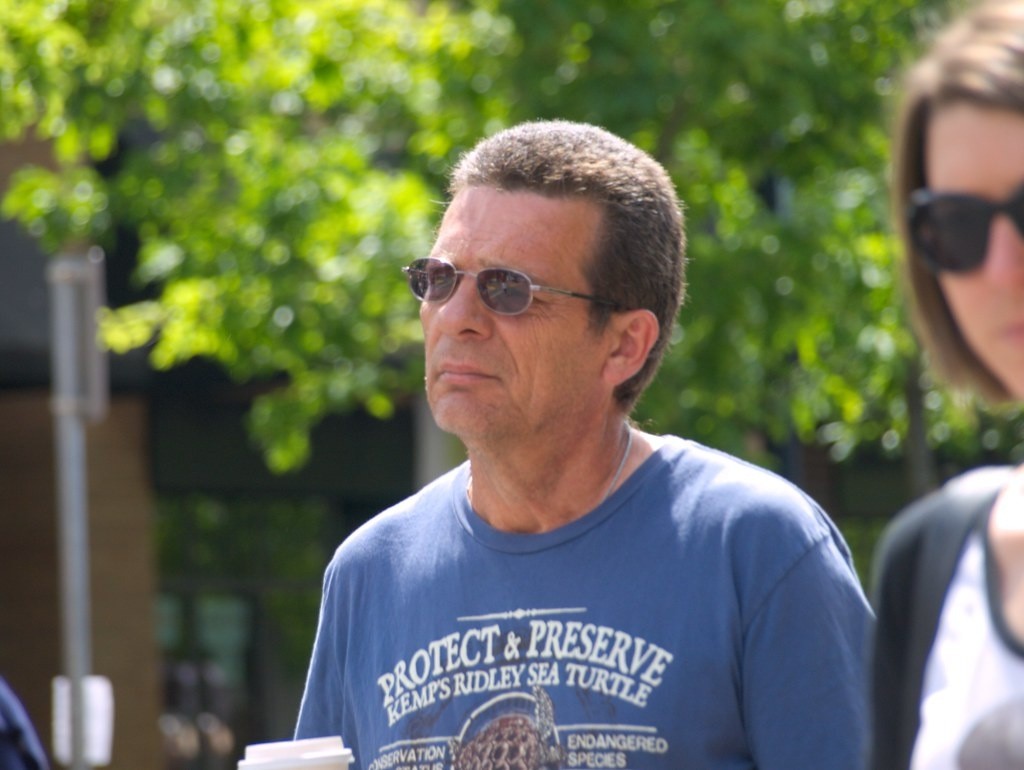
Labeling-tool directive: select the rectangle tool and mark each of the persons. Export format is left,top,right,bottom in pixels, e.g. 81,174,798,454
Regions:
867,0,1024,769
293,123,877,770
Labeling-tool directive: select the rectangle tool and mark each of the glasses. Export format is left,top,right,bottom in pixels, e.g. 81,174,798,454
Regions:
903,183,1024,272
402,256,628,317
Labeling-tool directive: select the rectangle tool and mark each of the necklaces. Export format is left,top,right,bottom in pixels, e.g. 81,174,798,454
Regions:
466,420,632,505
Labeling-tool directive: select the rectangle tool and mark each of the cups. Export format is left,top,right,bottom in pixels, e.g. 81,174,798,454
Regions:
236,735,355,770
